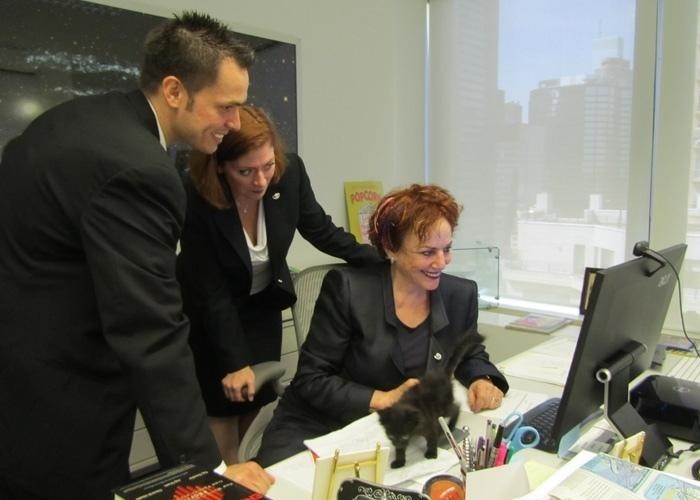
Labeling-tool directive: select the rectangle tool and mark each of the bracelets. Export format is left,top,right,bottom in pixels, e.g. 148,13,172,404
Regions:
484,374,494,381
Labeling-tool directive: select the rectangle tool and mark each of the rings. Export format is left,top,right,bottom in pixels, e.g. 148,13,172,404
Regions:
492,397,498,403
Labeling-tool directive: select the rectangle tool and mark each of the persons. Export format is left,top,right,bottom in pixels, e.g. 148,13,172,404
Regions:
172,104,392,455
255,183,509,466
2,11,275,499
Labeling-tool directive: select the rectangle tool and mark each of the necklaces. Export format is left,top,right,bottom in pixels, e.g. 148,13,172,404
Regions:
233,195,252,214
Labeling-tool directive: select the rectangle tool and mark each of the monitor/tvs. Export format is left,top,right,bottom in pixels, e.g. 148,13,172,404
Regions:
552,243,691,471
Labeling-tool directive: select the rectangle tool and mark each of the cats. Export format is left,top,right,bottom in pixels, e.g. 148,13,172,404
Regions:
376,335,485,469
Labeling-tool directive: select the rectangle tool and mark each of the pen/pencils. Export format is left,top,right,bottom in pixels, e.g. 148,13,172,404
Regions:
438,415,515,471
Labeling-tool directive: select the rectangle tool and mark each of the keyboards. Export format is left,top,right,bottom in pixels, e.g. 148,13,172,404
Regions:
502,396,561,448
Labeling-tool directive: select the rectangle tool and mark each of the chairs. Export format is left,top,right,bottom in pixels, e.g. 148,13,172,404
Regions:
228,261,351,463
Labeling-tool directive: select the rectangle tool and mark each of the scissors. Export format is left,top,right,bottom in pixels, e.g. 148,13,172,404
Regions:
504,412,541,452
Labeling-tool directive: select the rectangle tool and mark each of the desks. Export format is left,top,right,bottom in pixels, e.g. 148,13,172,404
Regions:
245,327,699,500
